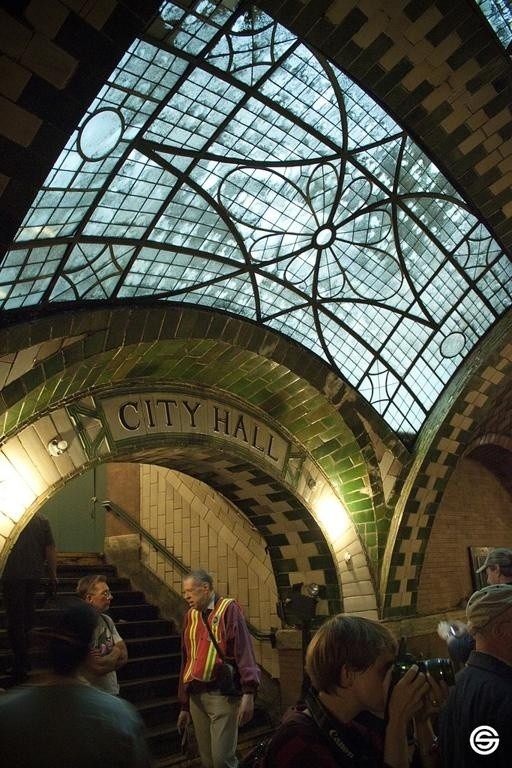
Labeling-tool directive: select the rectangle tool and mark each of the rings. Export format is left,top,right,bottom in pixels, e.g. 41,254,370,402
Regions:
431,694,441,706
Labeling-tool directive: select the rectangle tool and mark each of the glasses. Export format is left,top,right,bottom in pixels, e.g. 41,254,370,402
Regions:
86,591,112,599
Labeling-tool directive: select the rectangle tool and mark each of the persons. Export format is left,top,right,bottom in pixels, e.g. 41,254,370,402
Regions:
1,599,152,768
433,547,512,670
436,583,512,767
174,569,262,768
0,512,61,684
253,611,454,767
71,572,129,702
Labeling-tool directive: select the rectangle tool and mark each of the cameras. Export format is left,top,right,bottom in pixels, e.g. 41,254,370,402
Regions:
398,635,456,687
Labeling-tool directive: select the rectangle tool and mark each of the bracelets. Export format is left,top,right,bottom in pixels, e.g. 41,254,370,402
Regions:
444,630,453,640
413,736,440,757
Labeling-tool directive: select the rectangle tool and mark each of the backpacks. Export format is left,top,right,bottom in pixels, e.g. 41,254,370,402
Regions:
239,739,274,767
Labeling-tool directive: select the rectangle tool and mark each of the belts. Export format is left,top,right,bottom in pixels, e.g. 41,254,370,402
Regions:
185,679,225,696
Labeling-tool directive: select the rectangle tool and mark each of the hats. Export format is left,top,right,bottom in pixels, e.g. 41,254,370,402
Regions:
466,582,512,631
475,547,511,575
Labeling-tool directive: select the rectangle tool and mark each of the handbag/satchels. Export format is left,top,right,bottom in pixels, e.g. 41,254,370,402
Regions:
214,657,263,697
